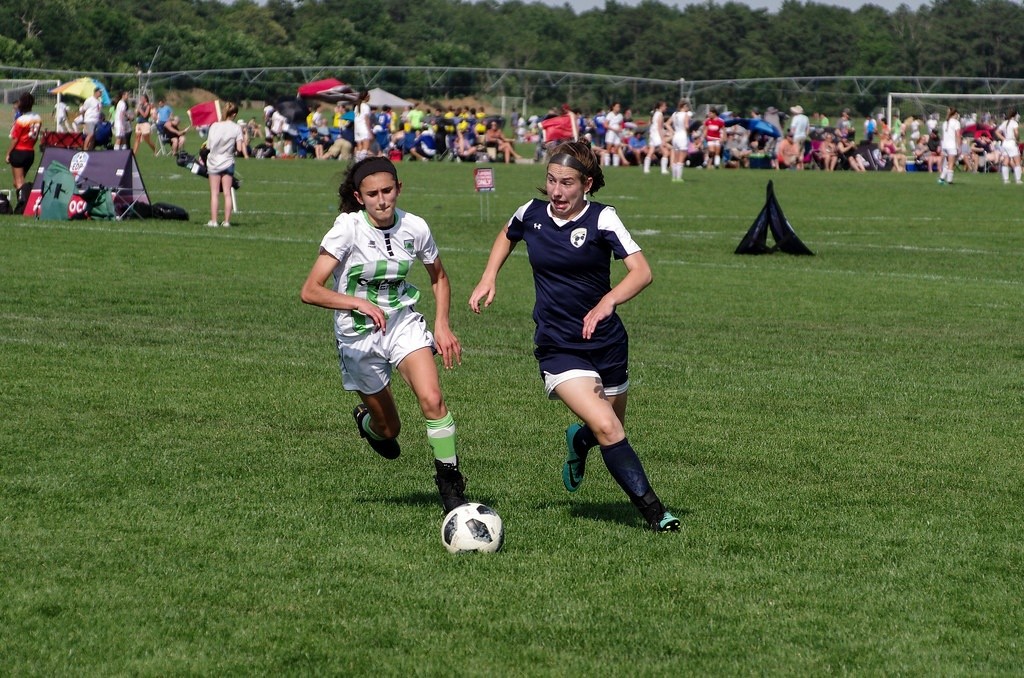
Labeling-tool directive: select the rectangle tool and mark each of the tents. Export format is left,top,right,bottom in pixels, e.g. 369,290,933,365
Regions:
736,181,817,254
22,148,152,220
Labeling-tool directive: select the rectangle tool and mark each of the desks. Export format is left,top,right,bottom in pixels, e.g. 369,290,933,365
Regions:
86,187,148,223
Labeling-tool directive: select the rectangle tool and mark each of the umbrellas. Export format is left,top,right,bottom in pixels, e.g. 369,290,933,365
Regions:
48,78,110,122
367,88,415,106
739,118,781,136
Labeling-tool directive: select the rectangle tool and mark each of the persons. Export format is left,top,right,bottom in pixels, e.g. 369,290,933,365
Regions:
205,102,243,227
6,93,41,214
526,103,1023,193
134,94,157,153
468,140,683,534
370,104,526,163
52,96,71,133
108,101,116,127
94,112,114,150
255,90,372,162
248,117,263,138
72,114,83,131
115,90,135,150
302,156,466,515
237,119,250,158
12,99,21,120
150,100,186,154
79,87,101,151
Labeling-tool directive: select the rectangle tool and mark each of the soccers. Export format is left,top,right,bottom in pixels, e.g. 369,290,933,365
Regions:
441,502,505,552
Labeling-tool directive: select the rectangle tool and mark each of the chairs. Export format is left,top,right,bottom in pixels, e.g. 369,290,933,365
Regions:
153,120,180,158
972,144,1002,174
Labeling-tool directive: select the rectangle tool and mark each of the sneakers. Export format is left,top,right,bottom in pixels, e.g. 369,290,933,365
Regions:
562,424,587,492
353,404,400,459
434,457,465,514
649,511,680,533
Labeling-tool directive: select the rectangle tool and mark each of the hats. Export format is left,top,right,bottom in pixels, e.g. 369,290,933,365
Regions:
94,88,101,92
790,105,803,114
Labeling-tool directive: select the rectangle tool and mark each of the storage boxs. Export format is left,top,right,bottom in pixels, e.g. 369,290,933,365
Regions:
905,161,916,171
748,153,772,169
390,150,402,161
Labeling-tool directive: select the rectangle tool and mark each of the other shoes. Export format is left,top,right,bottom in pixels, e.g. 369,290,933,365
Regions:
207,220,219,226
1017,180,1023,184
672,179,683,182
938,178,945,183
1004,180,1011,183
221,221,230,226
